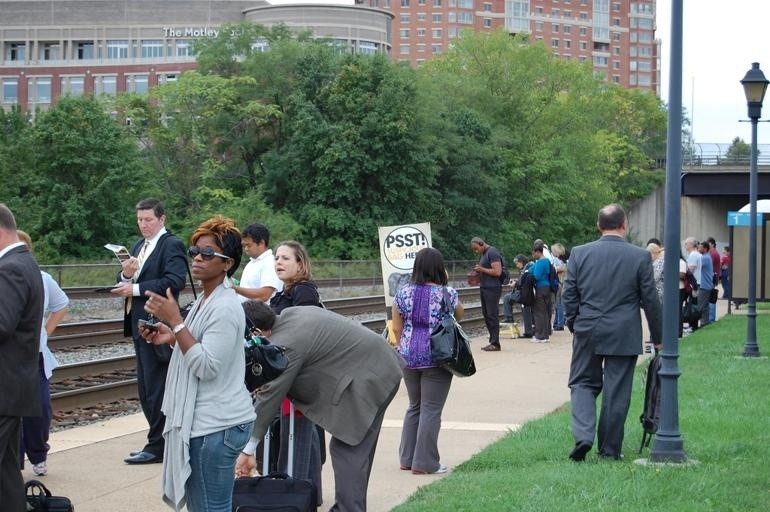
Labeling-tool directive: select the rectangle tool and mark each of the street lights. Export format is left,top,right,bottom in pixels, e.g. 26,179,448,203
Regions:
738,61,770,359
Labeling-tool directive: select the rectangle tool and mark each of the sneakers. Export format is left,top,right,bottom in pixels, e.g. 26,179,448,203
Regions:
518,327,564,343
501,317,514,323
481,345,501,350
400,463,448,474
33,460,47,475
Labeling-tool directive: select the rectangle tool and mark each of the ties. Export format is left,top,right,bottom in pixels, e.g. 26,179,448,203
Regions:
126,242,149,316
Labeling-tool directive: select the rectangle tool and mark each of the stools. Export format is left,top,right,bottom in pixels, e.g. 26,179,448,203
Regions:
499,322,521,338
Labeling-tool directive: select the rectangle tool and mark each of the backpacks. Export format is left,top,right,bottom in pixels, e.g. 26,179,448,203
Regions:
488,249,510,285
548,264,560,293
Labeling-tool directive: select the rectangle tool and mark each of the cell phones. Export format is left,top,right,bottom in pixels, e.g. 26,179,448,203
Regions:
137,319,159,334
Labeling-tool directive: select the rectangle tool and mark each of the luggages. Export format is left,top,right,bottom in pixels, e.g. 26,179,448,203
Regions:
231,400,318,511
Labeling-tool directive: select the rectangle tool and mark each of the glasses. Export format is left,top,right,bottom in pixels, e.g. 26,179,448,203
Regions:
187,245,231,260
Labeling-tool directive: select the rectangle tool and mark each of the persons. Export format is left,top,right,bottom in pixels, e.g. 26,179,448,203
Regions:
110,197,409,511
0,203,43,512
392,247,464,474
561,203,663,461
16,229,70,476
470,236,731,351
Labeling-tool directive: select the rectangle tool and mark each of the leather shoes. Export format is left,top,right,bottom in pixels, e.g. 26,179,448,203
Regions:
569,440,592,461
124,452,162,464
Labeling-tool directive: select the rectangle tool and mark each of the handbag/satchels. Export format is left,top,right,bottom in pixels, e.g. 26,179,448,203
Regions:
682,302,700,323
639,348,662,455
25,480,74,512
708,289,719,304
245,335,291,392
148,302,194,361
430,313,476,376
